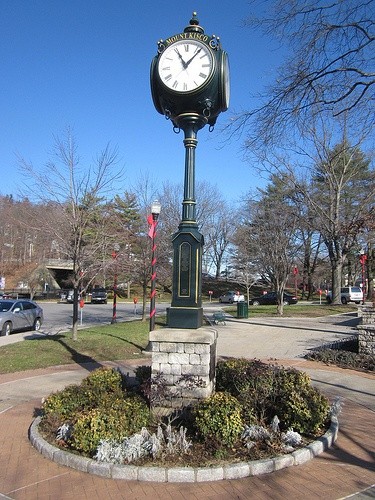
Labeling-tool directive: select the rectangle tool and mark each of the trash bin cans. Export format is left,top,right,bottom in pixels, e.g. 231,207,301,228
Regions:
237,301,248,318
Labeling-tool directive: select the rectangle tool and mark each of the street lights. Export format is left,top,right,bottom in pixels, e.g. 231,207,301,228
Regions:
358,249,368,306
142,199,162,353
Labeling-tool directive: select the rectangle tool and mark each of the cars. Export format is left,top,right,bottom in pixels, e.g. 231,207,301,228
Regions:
219,291,244,303
68,291,81,303
249,291,298,306
90,291,108,304
0,299,44,336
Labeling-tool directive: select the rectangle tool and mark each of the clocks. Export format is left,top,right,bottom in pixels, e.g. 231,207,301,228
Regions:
156,38,218,98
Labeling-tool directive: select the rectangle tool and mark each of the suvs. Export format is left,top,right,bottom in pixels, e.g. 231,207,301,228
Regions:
325,286,364,305
